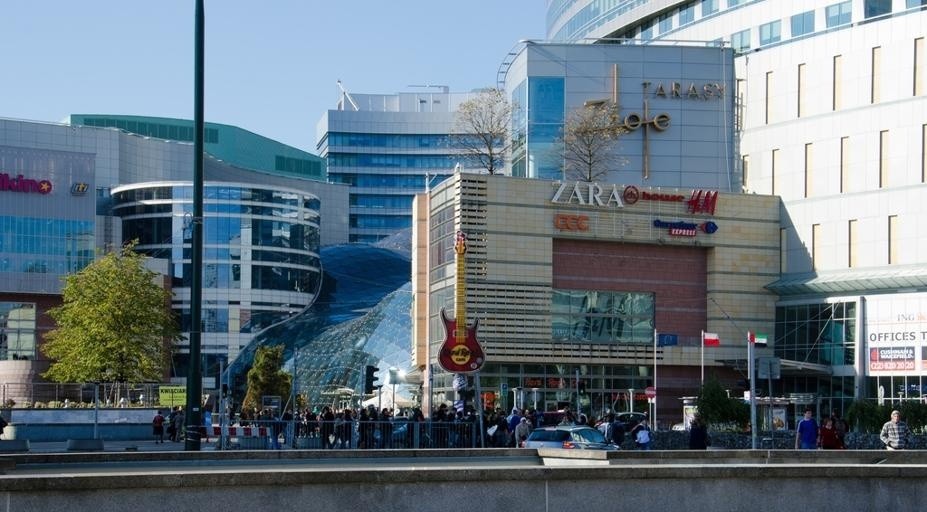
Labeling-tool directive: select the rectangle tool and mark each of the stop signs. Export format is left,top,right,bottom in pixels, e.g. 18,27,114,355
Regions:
644,387,655,398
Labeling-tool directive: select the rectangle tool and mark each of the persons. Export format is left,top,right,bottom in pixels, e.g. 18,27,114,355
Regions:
879,410,911,450
204,405,428,449
830,407,841,430
815,416,843,448
685,412,709,451
153,407,185,444
0,398,17,434
559,407,651,449
794,407,820,448
434,403,543,449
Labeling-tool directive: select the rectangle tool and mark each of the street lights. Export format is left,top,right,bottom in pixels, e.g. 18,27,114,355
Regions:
725,389,732,398
627,389,634,419
377,384,383,418
511,386,518,408
530,387,538,411
896,392,903,406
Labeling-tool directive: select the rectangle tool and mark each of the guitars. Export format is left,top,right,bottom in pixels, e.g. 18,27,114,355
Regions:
438,231,485,373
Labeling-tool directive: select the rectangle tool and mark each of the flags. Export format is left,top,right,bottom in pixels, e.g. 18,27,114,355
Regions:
655,333,679,347
702,332,720,347
748,332,768,344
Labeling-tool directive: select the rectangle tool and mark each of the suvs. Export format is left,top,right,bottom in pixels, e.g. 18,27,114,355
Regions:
595,412,653,439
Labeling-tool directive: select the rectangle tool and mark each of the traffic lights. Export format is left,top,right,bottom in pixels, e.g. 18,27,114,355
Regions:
578,382,585,396
221,384,227,399
364,365,379,394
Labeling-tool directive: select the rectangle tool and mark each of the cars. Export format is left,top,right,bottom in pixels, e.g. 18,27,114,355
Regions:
520,425,622,450
372,416,408,444
672,421,684,431
527,410,579,427
